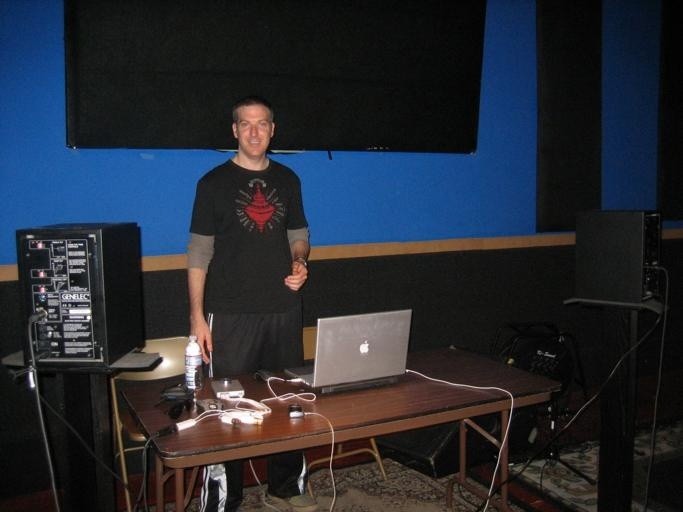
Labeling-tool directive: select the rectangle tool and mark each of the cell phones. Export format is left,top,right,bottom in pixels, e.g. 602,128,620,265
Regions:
289,405,303,418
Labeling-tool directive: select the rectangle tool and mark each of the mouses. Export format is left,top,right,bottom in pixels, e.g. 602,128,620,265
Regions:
254,368,275,381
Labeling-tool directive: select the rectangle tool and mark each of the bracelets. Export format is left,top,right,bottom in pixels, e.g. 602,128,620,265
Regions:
292,255,308,268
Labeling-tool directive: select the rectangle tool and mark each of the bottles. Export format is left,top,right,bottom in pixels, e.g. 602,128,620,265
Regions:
185,335,203,391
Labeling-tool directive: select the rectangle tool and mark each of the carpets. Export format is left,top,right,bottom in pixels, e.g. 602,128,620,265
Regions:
511,424,683,511
149,420,537,511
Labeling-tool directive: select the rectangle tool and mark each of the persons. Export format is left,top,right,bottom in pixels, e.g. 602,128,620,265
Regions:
183,95,312,512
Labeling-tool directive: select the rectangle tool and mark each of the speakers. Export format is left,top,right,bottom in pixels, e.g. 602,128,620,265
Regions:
575,210,660,302
373,418,460,479
15,223,145,365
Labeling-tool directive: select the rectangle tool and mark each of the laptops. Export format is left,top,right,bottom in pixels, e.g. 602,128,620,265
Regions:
285,309,413,394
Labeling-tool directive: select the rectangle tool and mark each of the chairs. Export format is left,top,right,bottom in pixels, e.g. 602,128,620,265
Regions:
303,326,387,505
110,336,188,512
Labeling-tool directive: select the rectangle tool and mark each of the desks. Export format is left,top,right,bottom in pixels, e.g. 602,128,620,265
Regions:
120,347,563,512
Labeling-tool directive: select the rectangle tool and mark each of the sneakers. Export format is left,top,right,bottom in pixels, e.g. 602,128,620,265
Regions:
268,488,318,512
